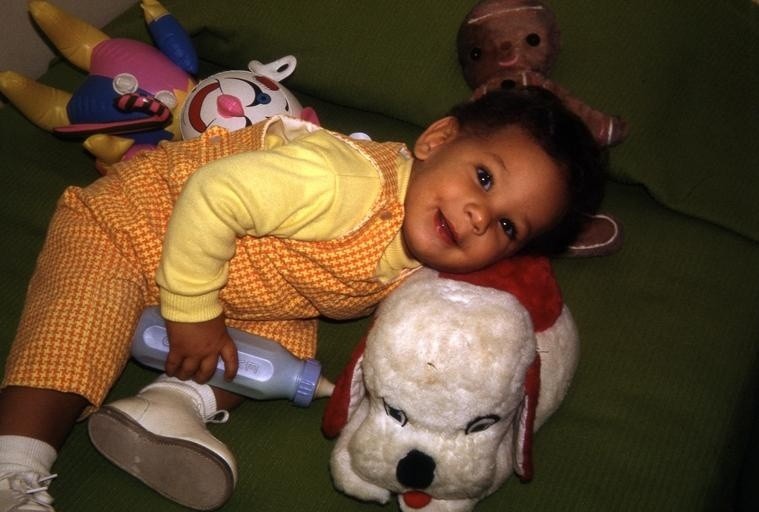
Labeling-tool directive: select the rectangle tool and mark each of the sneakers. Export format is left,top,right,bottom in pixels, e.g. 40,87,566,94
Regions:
1,467,61,512
85,386,239,512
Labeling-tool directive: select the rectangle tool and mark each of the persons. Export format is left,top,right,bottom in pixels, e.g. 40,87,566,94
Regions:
0,86,609,512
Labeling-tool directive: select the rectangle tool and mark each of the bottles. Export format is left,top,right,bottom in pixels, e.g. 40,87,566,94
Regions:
131,305,335,406
